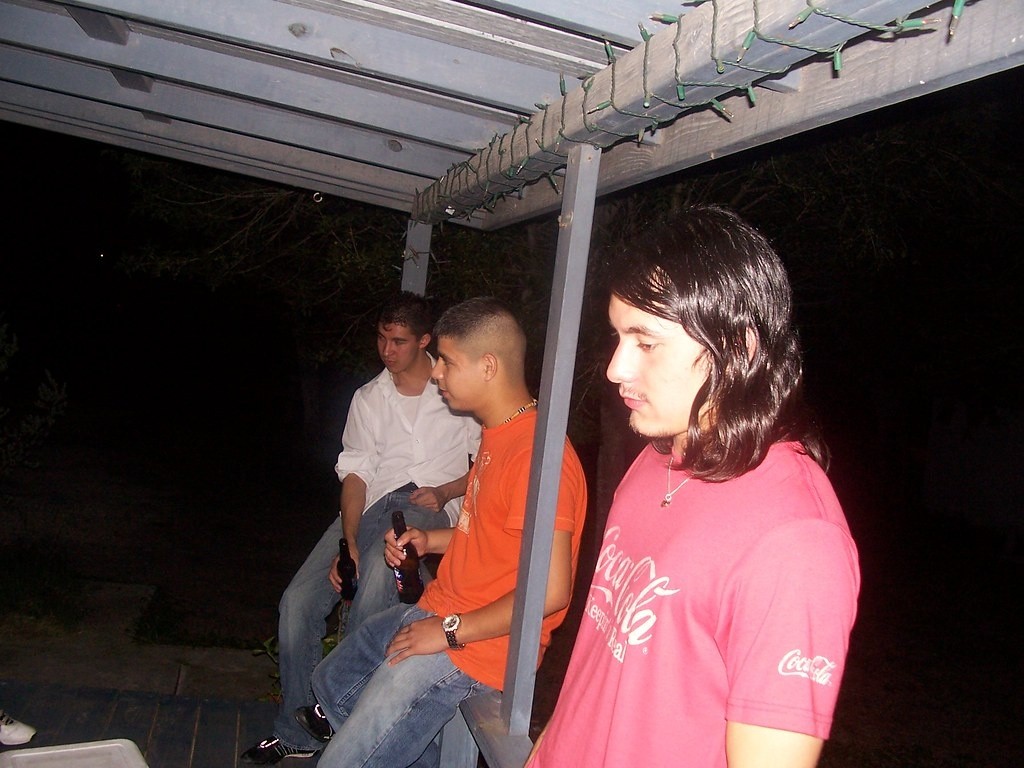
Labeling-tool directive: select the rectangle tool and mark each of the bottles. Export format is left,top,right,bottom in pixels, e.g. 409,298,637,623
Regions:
336,538,358,600
390,509,423,604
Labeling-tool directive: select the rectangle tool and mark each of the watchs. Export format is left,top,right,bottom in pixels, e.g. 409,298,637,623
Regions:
442,613,467,652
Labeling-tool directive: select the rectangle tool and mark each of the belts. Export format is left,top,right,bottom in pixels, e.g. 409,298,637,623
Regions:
393,483,419,493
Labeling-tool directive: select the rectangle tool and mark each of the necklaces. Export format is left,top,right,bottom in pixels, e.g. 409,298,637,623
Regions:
660,457,698,507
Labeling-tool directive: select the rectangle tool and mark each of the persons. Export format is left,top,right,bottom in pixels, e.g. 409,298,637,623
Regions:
522,206,859,768
310,298,587,768
240,291,484,768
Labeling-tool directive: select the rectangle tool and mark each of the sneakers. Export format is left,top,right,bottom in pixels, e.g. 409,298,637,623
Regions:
240,736,317,763
0,709,37,745
294,704,335,742
